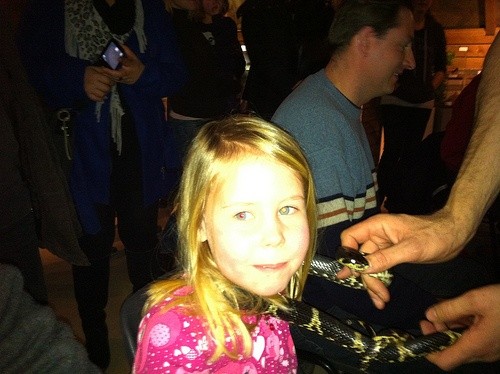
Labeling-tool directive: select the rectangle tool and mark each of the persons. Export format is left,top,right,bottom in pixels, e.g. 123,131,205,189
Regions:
130,115,318,374
0,0,500,374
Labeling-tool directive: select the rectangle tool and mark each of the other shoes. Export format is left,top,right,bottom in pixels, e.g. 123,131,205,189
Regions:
87,339,111,371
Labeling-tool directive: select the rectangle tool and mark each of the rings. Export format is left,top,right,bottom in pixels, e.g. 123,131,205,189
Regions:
122,71,127,77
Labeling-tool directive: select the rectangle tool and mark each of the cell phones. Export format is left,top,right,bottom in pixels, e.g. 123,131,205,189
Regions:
94,39,126,70
